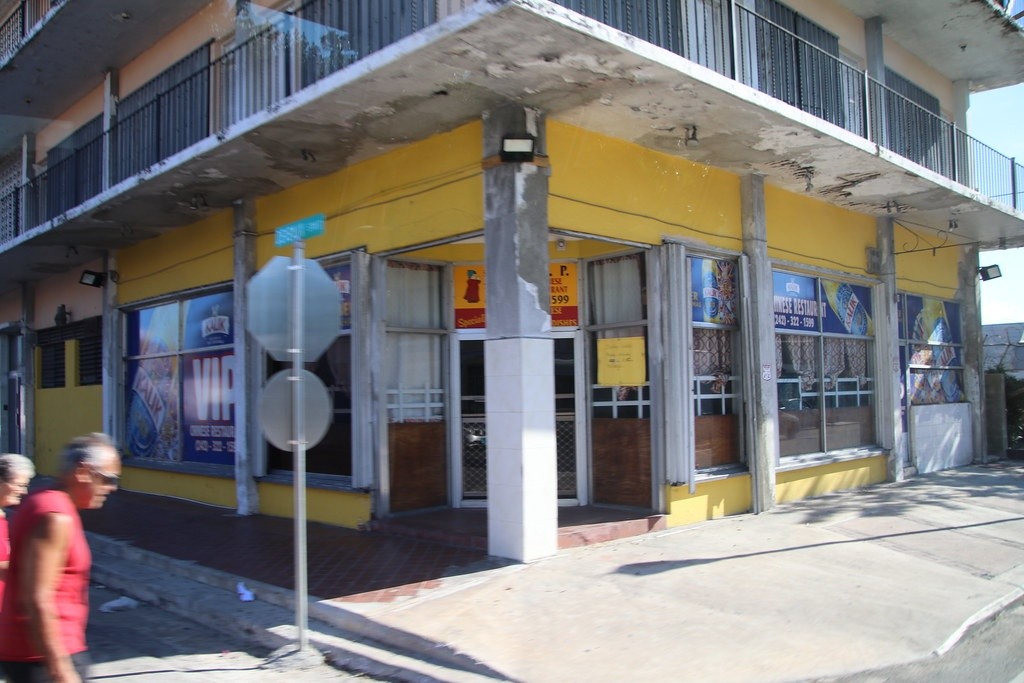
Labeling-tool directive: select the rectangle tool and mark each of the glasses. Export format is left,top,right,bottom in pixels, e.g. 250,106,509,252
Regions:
88,469,123,484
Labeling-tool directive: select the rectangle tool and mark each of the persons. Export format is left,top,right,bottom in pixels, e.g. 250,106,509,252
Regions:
0,432,123,683
